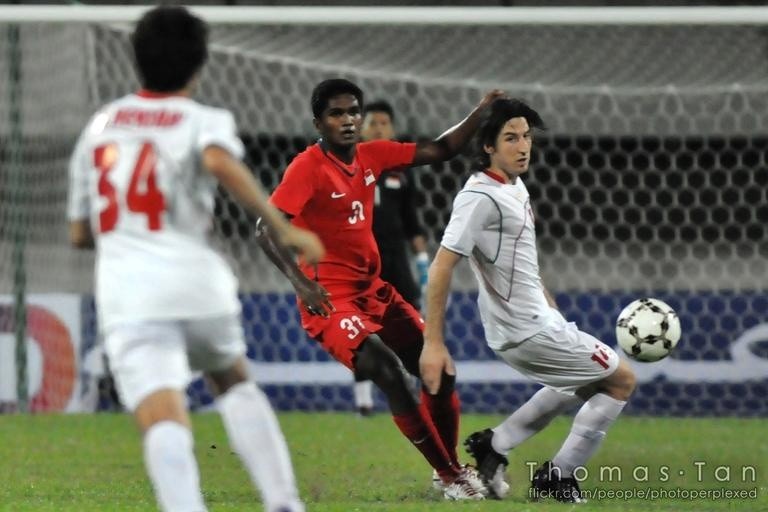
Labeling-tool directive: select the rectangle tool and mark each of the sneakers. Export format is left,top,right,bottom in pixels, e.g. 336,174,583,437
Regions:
528,461,588,504
432,463,489,500
464,428,510,500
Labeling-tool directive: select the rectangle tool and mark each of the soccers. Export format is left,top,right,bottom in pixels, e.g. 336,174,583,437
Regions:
615,298,682,363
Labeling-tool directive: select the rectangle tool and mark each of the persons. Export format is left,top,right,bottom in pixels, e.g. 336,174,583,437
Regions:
347,97,432,418
413,97,640,500
255,74,512,504
63,7,330,511
